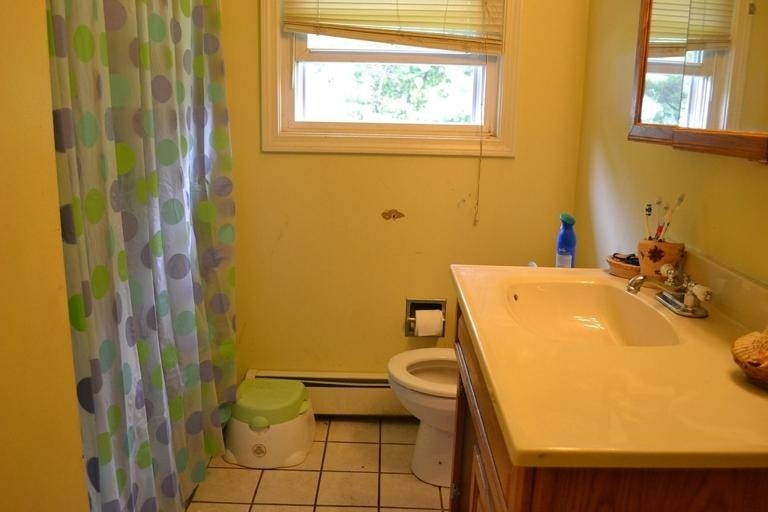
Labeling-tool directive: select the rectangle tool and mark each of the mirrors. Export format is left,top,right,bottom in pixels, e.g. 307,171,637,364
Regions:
627,0,768,161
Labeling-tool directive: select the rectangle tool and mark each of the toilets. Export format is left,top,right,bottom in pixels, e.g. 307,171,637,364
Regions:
387,347,458,488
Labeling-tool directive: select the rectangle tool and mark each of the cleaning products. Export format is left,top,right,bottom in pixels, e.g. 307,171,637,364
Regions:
556,211,577,267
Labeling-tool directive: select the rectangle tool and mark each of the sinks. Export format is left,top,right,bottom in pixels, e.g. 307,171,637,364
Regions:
504,275,679,347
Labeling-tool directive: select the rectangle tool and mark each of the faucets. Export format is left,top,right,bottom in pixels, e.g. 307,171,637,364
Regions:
625,267,685,298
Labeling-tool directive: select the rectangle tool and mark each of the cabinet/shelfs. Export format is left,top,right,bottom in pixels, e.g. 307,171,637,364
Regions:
446,295,767,511
